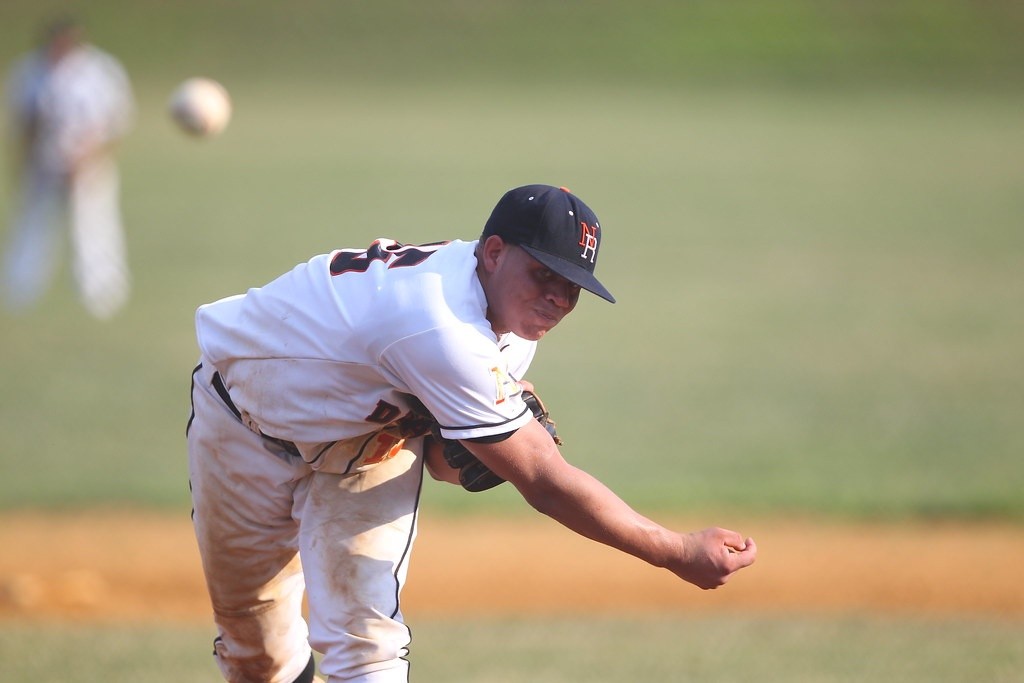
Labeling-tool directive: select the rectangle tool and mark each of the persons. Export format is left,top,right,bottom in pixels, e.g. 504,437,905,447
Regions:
4,17,138,317
185,183,756,683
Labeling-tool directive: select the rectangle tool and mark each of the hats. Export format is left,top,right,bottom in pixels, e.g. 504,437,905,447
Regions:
482,184,617,303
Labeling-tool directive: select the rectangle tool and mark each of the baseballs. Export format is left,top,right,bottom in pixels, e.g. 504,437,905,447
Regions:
175,77,231,134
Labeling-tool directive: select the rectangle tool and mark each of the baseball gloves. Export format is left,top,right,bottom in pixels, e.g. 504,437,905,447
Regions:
405,389,560,492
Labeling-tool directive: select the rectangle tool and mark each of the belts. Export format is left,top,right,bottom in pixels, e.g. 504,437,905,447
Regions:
209,370,302,458
